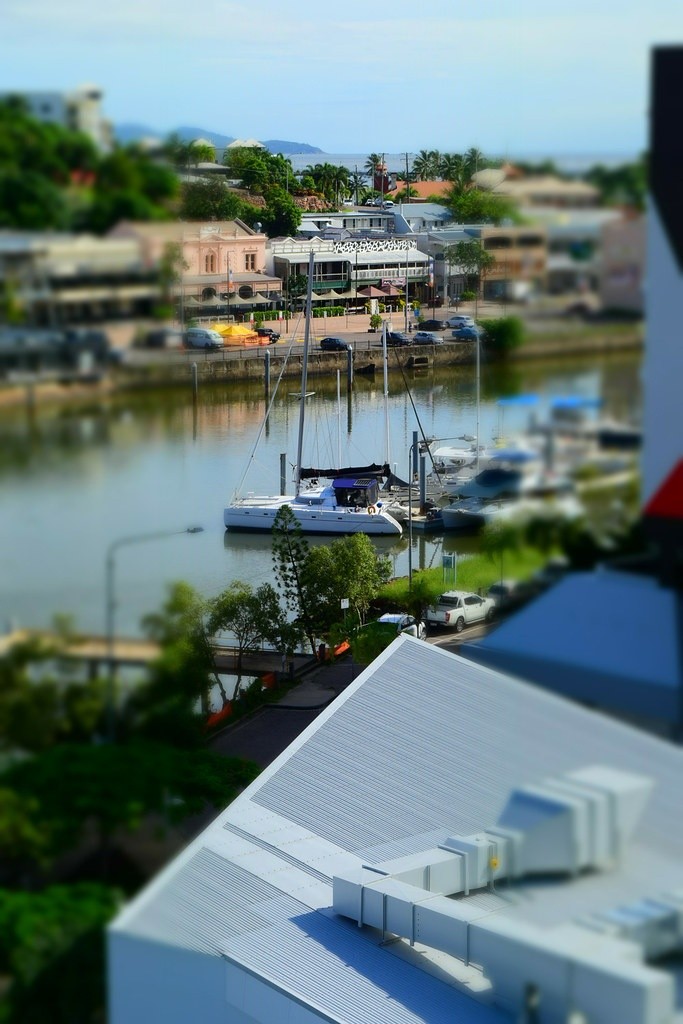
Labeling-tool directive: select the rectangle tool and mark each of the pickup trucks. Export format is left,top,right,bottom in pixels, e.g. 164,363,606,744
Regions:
420,589,496,634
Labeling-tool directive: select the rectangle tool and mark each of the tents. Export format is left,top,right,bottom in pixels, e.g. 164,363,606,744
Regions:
175,291,290,317
293,284,406,311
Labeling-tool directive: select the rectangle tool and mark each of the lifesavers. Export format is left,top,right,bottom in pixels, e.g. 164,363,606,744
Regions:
368,506,376,514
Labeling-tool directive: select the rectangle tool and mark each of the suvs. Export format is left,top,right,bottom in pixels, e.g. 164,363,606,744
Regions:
381,331,413,347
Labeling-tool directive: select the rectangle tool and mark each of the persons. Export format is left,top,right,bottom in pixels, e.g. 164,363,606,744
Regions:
349,490,363,504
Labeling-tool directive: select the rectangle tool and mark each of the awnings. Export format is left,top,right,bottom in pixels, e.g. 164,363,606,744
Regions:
210,323,258,338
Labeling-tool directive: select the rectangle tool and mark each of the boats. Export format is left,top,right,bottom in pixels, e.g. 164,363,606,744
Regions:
220,249,643,539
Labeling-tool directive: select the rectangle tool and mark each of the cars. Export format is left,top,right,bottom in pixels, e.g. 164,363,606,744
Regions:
255,328,280,344
446,315,475,329
484,578,521,607
376,612,427,643
451,327,480,342
413,332,445,345
415,320,445,332
320,337,352,351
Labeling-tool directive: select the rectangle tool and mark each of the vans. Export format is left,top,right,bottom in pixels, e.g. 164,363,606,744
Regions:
185,328,224,350
